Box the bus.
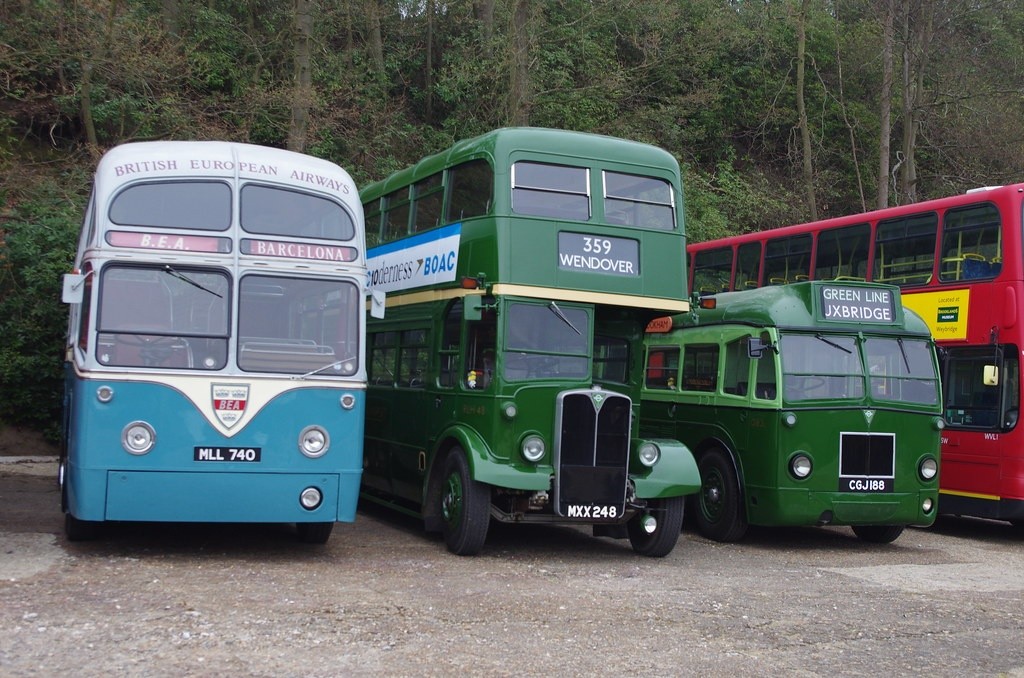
[651,276,944,546]
[359,127,703,559]
[637,181,1024,536]
[58,140,385,545]
